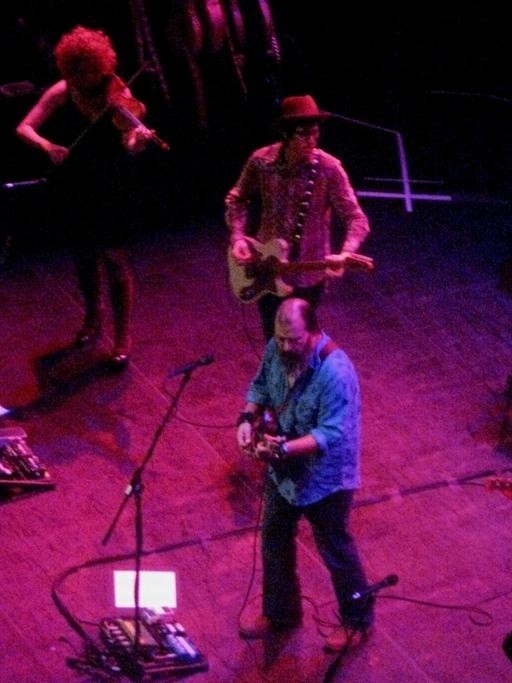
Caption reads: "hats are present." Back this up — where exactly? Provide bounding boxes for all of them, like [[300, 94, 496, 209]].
[[277, 93, 333, 120]]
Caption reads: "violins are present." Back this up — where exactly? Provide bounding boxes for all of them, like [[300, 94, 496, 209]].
[[68, 66, 172, 154]]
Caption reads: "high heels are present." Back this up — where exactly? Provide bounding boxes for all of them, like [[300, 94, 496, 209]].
[[76, 311, 108, 346], [108, 333, 132, 369]]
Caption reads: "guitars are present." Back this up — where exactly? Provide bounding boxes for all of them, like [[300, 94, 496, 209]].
[[228, 238, 373, 307], [239, 408, 286, 461]]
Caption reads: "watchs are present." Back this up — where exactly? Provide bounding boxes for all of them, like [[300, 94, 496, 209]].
[[238, 410, 253, 425]]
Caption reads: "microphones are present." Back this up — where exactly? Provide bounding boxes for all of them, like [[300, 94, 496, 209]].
[[167, 352, 216, 376], [351, 572, 400, 598]]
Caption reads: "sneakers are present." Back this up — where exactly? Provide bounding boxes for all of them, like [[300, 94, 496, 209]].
[[242, 613, 278, 638], [327, 621, 371, 649]]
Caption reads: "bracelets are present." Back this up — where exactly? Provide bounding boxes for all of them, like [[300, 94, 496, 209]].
[[274, 444, 288, 460], [238, 411, 252, 424]]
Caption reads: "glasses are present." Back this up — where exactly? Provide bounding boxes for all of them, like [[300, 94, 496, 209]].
[[292, 129, 322, 139]]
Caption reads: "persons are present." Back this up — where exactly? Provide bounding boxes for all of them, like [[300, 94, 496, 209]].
[[236, 297, 368, 654], [16, 25, 156, 363], [224, 95, 370, 342]]
